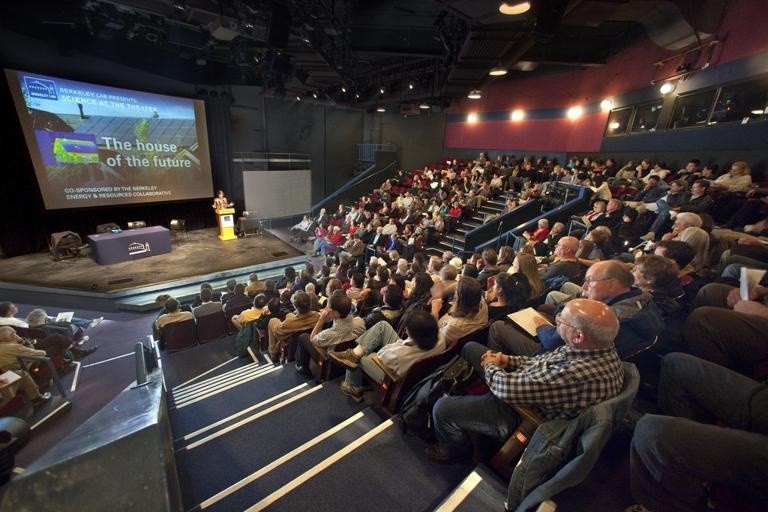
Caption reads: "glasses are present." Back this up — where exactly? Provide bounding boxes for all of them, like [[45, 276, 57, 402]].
[[555, 313, 575, 330], [584, 278, 613, 287]]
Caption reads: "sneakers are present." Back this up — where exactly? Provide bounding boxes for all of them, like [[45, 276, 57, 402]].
[[90, 316, 103, 328], [78, 335, 89, 346], [425, 448, 450, 463], [341, 381, 365, 403]]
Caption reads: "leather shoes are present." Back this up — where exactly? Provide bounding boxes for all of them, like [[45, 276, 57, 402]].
[[33, 392, 53, 409], [73, 346, 97, 360], [295, 364, 310, 378]]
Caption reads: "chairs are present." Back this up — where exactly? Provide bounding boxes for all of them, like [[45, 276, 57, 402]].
[[157, 319, 196, 350], [306, 338, 358, 380], [479, 365, 640, 470], [621, 335, 660, 360], [196, 312, 224, 345], [225, 303, 253, 335], [654, 290, 685, 308], [456, 319, 492, 345], [369, 343, 457, 409]]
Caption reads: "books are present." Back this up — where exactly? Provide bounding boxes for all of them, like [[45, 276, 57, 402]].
[[0, 369, 23, 390], [55, 311, 75, 324], [504, 304, 556, 344]]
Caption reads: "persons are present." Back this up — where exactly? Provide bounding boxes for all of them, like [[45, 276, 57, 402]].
[[476, 247, 501, 291], [638, 159, 672, 186], [719, 237, 768, 282], [537, 235, 580, 287], [512, 218, 550, 249], [486, 259, 664, 359], [697, 213, 719, 251], [595, 225, 611, 238], [191, 282, 222, 308], [152, 294, 195, 336], [221, 249, 443, 404], [508, 252, 545, 300], [654, 179, 688, 213], [661, 211, 703, 243], [639, 178, 714, 242], [543, 254, 679, 307], [0, 299, 29, 329], [720, 186, 768, 236], [674, 225, 710, 277], [211, 189, 234, 235], [0, 326, 101, 373], [288, 156, 394, 259], [615, 159, 636, 181], [461, 263, 480, 280], [421, 296, 626, 465], [589, 174, 612, 211], [576, 229, 613, 268], [587, 197, 624, 227], [438, 264, 458, 288], [442, 250, 454, 266], [621, 350, 768, 512], [496, 245, 516, 273], [519, 244, 535, 256], [563, 200, 606, 239], [590, 158, 606, 173], [465, 252, 482, 267], [0, 368, 52, 413], [487, 271, 533, 321], [632, 175, 668, 204], [601, 159, 618, 181], [527, 221, 566, 257], [574, 238, 595, 260], [672, 178, 713, 215], [486, 152, 589, 218], [686, 159, 720, 186], [634, 159, 651, 182], [449, 256, 463, 270], [193, 287, 223, 320], [612, 208, 638, 247], [688, 281, 768, 369], [25, 307, 105, 347], [671, 159, 702, 180], [653, 239, 698, 288], [712, 160, 753, 208], [442, 275, 489, 348], [393, 150, 486, 258]]
